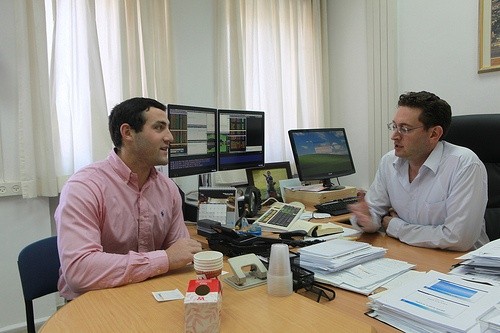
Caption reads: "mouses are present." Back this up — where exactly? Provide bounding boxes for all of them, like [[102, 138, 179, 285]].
[[289, 201, 306, 210]]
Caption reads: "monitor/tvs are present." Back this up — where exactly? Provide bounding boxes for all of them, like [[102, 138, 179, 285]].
[[288, 128, 356, 191], [165, 103, 265, 178]]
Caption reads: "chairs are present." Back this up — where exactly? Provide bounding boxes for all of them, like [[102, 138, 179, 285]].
[[18, 236, 60, 333], [444, 114, 500, 241]]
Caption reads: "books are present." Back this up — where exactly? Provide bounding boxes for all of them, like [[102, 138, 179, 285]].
[[287, 216, 343, 239]]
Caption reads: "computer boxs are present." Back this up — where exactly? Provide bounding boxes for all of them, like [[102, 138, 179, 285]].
[[284, 183, 358, 210]]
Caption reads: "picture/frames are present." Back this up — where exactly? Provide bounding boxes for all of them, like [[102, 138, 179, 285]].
[[478, 0, 500, 74], [246, 161, 293, 205]]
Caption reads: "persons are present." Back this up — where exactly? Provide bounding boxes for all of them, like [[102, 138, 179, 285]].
[[266, 170, 277, 191], [346, 90, 489, 252], [53, 97, 202, 305]]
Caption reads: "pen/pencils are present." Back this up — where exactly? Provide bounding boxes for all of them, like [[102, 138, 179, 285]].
[[449, 260, 466, 269]]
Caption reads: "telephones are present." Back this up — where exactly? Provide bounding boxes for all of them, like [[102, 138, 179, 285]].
[[198, 219, 282, 257]]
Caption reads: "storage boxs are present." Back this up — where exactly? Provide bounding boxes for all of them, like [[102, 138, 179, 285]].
[[183, 278, 222, 333]]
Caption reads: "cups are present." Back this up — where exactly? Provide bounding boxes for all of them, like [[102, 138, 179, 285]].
[[193, 250, 224, 281], [267, 243, 294, 297]]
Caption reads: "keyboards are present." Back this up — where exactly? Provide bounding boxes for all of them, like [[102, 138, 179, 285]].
[[252, 202, 303, 231], [313, 197, 358, 216]]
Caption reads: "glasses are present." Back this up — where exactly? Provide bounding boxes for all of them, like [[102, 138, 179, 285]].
[[387, 122, 424, 135]]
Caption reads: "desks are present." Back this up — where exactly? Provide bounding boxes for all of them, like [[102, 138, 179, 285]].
[[39, 206, 469, 333]]
